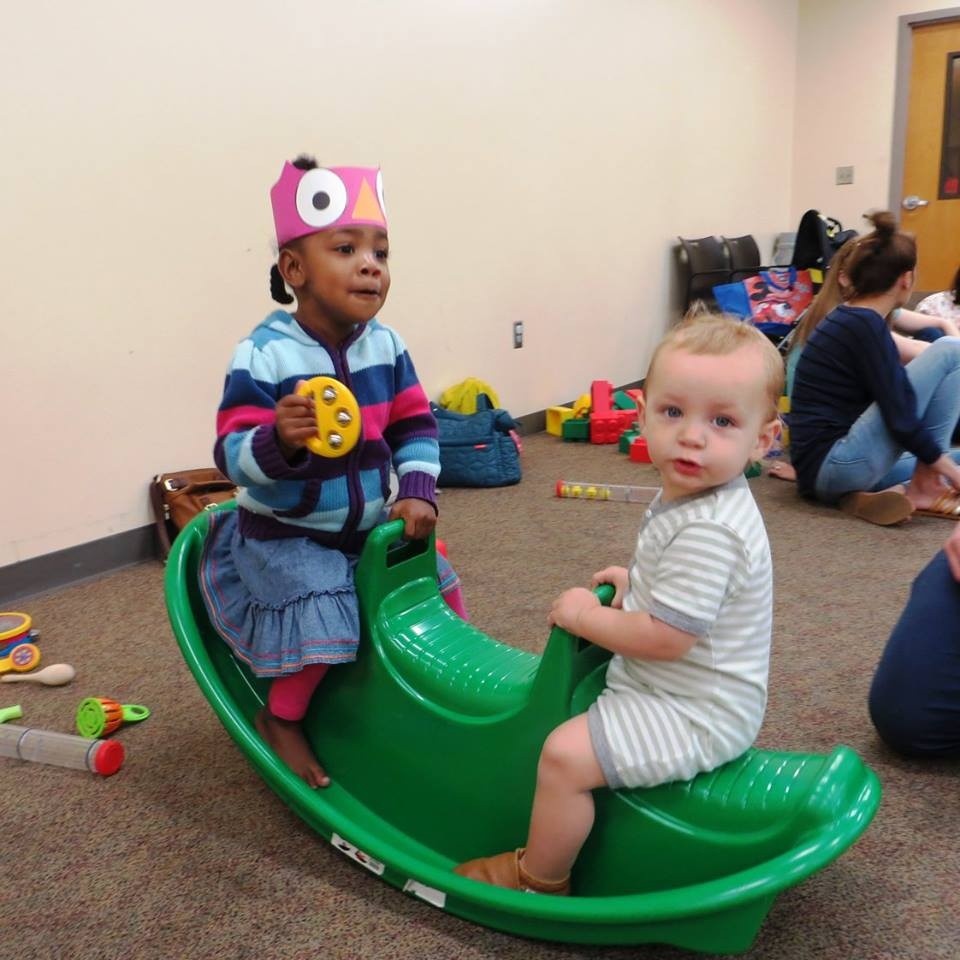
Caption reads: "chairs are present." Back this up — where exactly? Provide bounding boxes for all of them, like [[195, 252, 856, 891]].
[[679, 235, 791, 315]]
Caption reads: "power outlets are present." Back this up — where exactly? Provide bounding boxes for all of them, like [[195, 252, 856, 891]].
[[512, 321, 524, 349]]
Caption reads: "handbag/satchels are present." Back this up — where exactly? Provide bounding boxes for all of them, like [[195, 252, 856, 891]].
[[431, 393, 521, 487], [712, 265, 812, 335]]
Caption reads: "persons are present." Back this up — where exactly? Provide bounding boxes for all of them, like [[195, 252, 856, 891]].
[[451, 299, 784, 906], [868, 520, 960, 776], [788, 212, 960, 526], [198, 151, 464, 792]]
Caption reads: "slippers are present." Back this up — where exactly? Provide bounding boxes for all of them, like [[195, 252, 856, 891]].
[[908, 485, 960, 523], [844, 489, 913, 525]]
